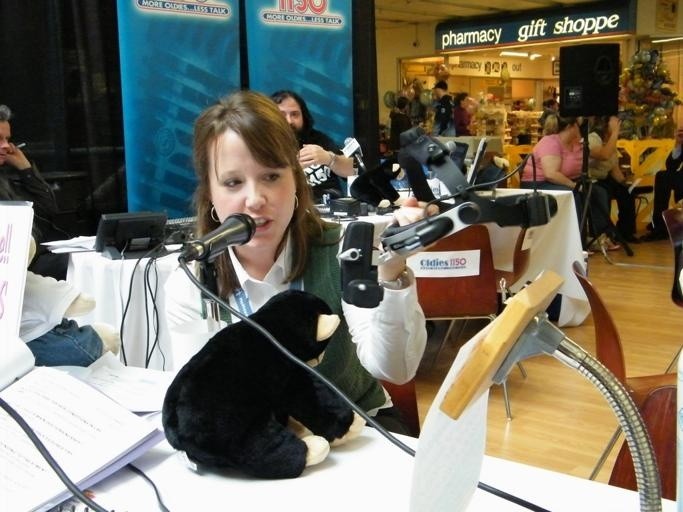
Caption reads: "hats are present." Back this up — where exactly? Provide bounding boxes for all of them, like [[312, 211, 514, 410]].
[[432, 80, 448, 90]]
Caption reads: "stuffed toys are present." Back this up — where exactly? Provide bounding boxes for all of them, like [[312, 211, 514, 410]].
[[350, 157, 405, 209], [158, 289, 364, 476]]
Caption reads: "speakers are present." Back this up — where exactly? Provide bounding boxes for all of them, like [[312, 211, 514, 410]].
[[558, 42, 621, 118]]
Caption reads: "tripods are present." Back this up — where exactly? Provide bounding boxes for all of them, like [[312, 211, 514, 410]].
[[571, 132, 634, 258]]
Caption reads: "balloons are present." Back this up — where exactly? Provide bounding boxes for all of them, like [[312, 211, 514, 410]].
[[383, 90, 396, 109], [434, 64, 450, 83], [616, 49, 678, 135]]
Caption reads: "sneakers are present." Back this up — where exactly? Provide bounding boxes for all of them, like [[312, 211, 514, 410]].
[[613, 234, 642, 244], [585, 234, 621, 256]]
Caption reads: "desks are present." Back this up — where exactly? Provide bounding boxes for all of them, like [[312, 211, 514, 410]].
[[0, 187, 677, 512], [433, 136, 504, 158]]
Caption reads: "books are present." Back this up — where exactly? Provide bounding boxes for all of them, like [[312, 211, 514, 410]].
[[0, 321, 164, 512]]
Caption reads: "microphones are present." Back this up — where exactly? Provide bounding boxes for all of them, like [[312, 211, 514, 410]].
[[176, 212, 257, 261], [340, 135, 369, 172], [445, 140, 458, 153]]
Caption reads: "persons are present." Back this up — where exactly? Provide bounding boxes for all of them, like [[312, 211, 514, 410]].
[[272, 92, 357, 201], [0, 104, 71, 282], [152, 90, 438, 436], [388, 79, 683, 256]]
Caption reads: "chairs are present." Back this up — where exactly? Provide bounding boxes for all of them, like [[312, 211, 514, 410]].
[[449, 136, 510, 190]]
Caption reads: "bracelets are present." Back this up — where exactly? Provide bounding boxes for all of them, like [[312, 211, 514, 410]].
[[327, 150, 336, 170]]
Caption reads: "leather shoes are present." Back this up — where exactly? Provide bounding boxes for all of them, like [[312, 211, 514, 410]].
[[640, 222, 670, 242]]
